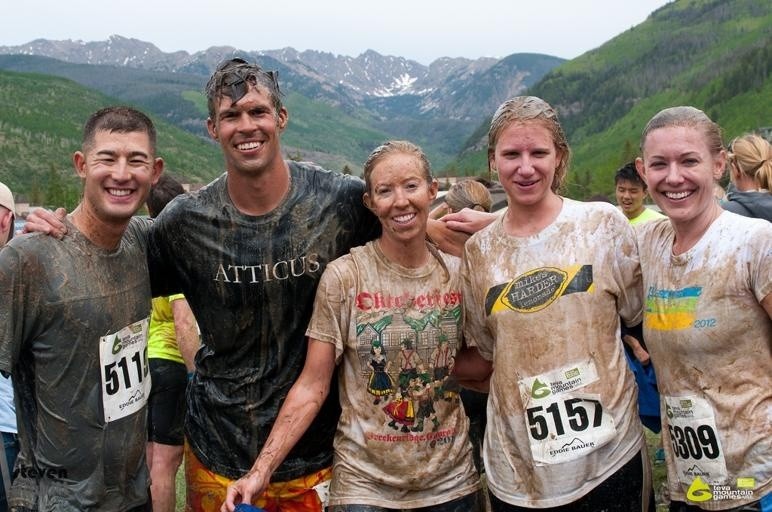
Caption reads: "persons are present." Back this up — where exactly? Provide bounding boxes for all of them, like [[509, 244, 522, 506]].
[[22, 53, 478, 512], [425, 177, 660, 433], [145, 167, 204, 510], [219, 135, 490, 511], [716, 132, 771, 221], [0, 104, 160, 512], [437, 98, 772, 509], [0, 183, 20, 512], [448, 92, 663, 511], [609, 160, 671, 229]]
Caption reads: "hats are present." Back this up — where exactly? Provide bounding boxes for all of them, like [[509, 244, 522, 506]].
[[0, 182, 17, 244]]
